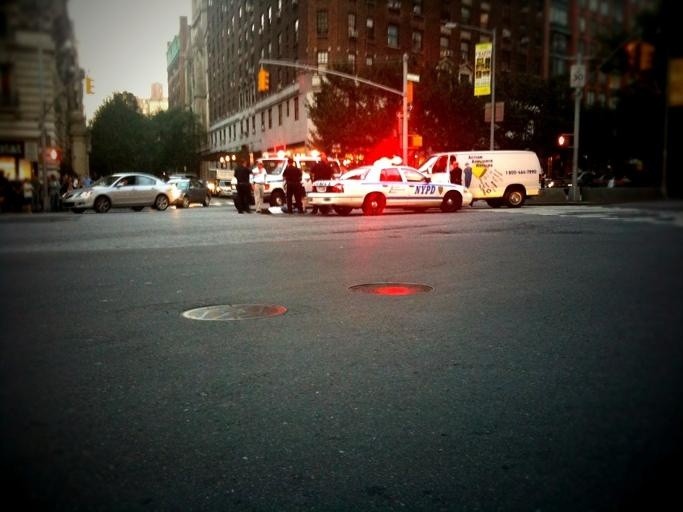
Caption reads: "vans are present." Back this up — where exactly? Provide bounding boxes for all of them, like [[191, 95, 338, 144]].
[[417, 149, 543, 208]]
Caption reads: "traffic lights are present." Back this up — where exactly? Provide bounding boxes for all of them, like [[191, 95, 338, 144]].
[[257, 70, 268, 93], [84, 76, 95, 95], [44, 147, 61, 161]]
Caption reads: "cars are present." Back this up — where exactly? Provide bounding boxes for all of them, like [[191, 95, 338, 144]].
[[60, 170, 174, 213], [167, 179, 210, 208], [306, 164, 474, 215], [214, 181, 231, 198], [230, 159, 309, 206]]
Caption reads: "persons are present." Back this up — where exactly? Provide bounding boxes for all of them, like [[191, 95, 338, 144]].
[[22, 174, 93, 215], [235, 152, 336, 214], [464, 163, 472, 188], [450, 161, 462, 185], [123, 179, 128, 184]]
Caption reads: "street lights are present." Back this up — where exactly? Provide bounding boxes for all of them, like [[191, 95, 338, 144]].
[[444, 20, 500, 151]]
